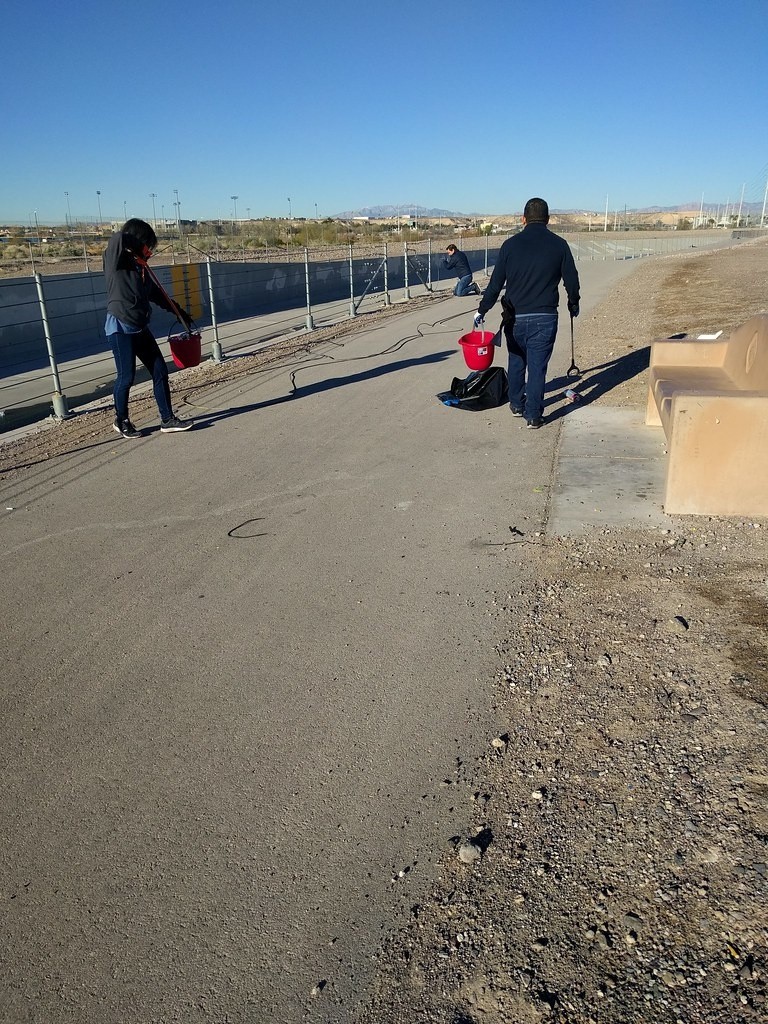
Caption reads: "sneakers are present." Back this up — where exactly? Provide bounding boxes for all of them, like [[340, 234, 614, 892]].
[[113, 416, 194, 439]]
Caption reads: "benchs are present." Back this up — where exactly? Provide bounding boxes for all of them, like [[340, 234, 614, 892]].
[[645, 313, 768, 518]]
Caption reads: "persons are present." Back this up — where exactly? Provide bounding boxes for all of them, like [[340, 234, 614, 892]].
[[442, 244, 481, 296], [104, 218, 194, 441], [472, 197, 582, 428]]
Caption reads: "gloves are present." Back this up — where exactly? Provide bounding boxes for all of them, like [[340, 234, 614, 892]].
[[473, 311, 485, 328], [567, 297, 581, 317]]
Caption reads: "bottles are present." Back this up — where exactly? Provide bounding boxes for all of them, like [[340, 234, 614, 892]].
[[564, 388, 581, 403]]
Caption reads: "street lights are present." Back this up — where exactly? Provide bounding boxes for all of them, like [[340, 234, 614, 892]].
[[28, 187, 324, 241]]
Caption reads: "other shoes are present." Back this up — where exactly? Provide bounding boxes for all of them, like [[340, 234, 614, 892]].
[[472, 282, 481, 295], [509, 401, 546, 429]]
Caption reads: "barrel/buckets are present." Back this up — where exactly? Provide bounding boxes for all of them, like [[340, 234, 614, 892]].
[[457, 316, 496, 371], [168, 318, 201, 370]]
